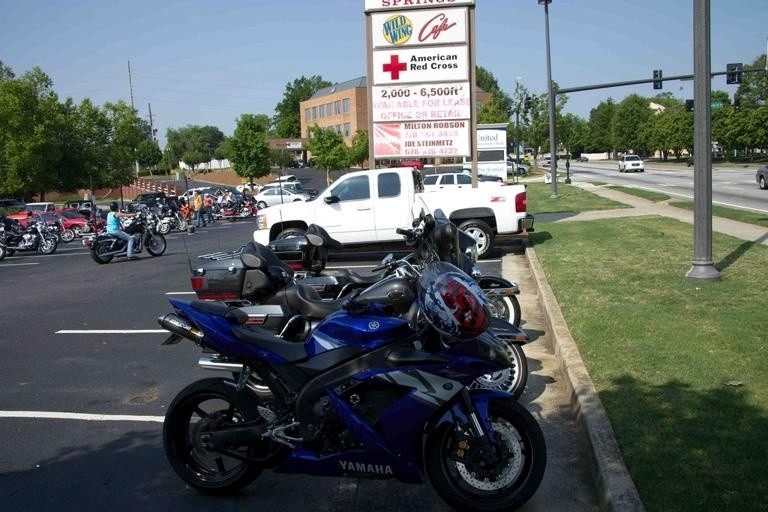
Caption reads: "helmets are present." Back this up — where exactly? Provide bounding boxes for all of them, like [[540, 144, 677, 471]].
[[110, 202, 118, 211], [417, 262, 491, 340]]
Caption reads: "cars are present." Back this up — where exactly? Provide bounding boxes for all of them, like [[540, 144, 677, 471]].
[[542, 156, 551, 167], [687, 154, 694, 167], [506, 160, 530, 177], [617, 154, 644, 173], [576, 157, 587, 162], [755, 163, 768, 189], [128, 175, 315, 223], [6, 200, 107, 239]]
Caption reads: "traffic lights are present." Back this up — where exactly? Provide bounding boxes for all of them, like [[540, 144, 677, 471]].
[[526, 96, 532, 109], [686, 99, 695, 112]]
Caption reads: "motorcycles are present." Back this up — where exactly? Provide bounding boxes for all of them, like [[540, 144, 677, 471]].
[[0, 211, 75, 262], [79, 197, 188, 249], [187, 194, 531, 400], [160, 295, 548, 510], [84, 212, 167, 264], [262, 207, 522, 331]]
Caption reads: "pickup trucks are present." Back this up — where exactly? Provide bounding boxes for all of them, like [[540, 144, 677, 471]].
[[252, 158, 535, 262]]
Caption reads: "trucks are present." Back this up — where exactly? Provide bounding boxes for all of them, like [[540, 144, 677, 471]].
[[711, 141, 723, 158]]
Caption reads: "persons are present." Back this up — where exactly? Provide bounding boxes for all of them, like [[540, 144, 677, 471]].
[[106, 201, 140, 261], [177, 188, 257, 227]]
